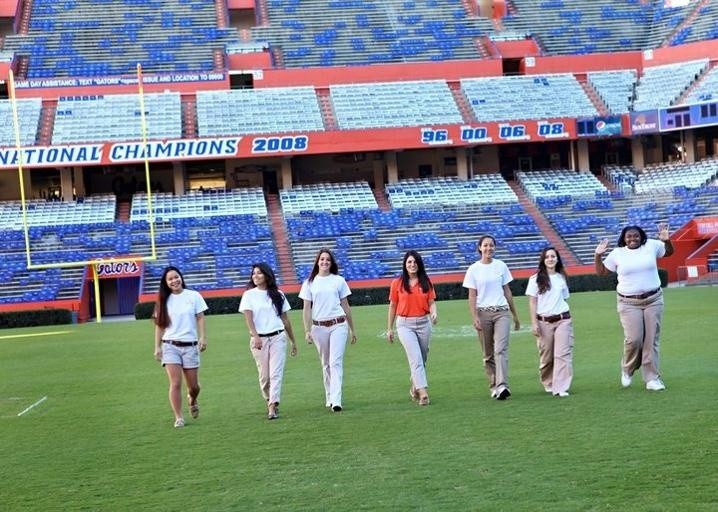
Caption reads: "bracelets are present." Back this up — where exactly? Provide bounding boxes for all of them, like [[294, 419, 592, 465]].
[[386, 328, 394, 335], [303, 328, 310, 334]]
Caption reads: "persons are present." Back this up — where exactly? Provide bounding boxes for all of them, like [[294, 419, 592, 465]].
[[151, 265, 210, 428], [461, 235, 521, 401], [297, 246, 358, 412], [386, 248, 439, 406], [524, 246, 575, 398], [594, 221, 676, 393], [238, 261, 297, 420]]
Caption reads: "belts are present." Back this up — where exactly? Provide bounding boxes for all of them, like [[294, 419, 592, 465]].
[[161, 341, 197, 346], [536, 311, 571, 324], [476, 305, 508, 313], [258, 330, 283, 337], [313, 318, 344, 327], [617, 288, 659, 299]]
